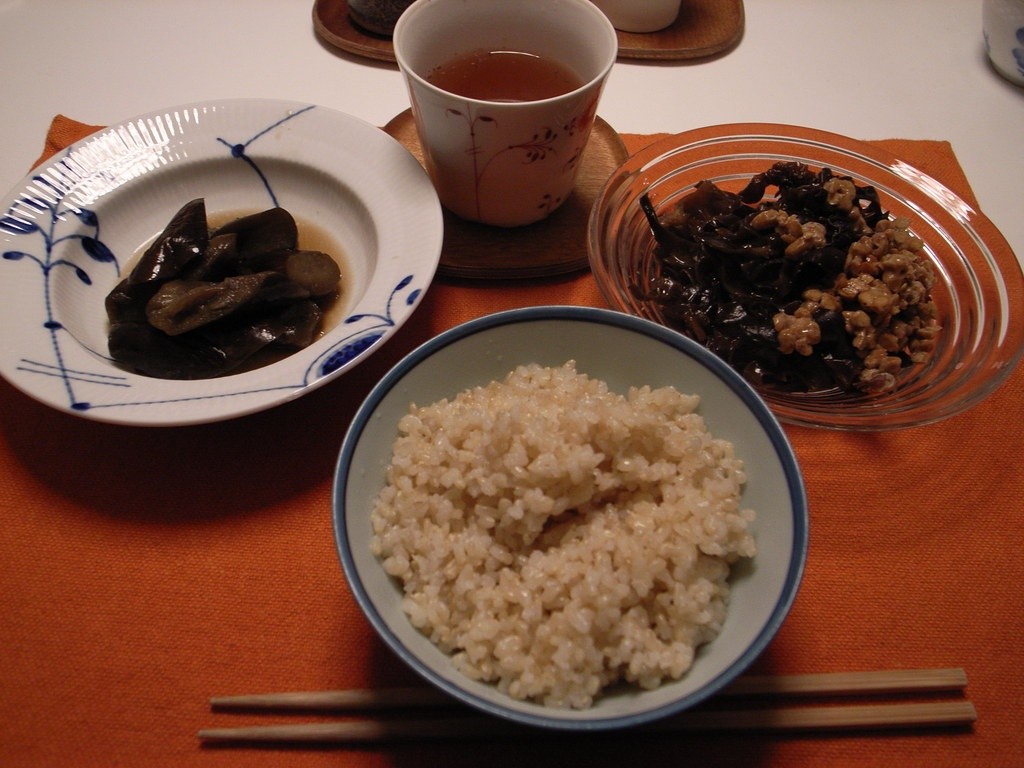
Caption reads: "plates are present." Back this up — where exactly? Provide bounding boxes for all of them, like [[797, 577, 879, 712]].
[[0, 95, 443, 427]]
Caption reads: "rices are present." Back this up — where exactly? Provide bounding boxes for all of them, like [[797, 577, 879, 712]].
[[370, 360, 756, 709]]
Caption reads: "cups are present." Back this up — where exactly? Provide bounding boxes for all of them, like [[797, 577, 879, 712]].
[[981, 0, 1024, 89], [589, 0, 682, 34], [347, 0, 416, 40], [393, 0, 618, 229]]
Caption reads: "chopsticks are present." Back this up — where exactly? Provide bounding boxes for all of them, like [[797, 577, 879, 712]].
[[197, 668, 979, 741]]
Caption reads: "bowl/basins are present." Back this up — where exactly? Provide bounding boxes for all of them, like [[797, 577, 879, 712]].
[[331, 303, 808, 733], [586, 122, 1024, 432]]
